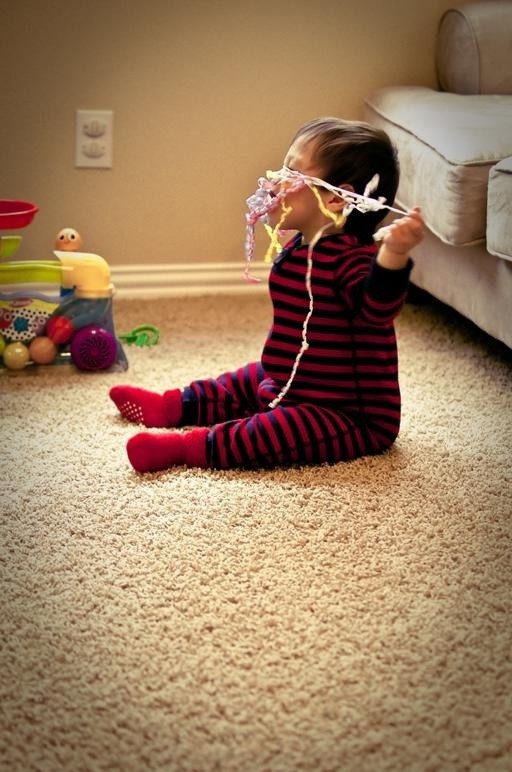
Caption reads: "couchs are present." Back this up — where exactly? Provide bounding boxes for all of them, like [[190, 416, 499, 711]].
[[364, 6, 512, 362]]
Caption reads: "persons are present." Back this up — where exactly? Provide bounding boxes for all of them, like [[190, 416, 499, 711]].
[[109, 115, 426, 473]]
